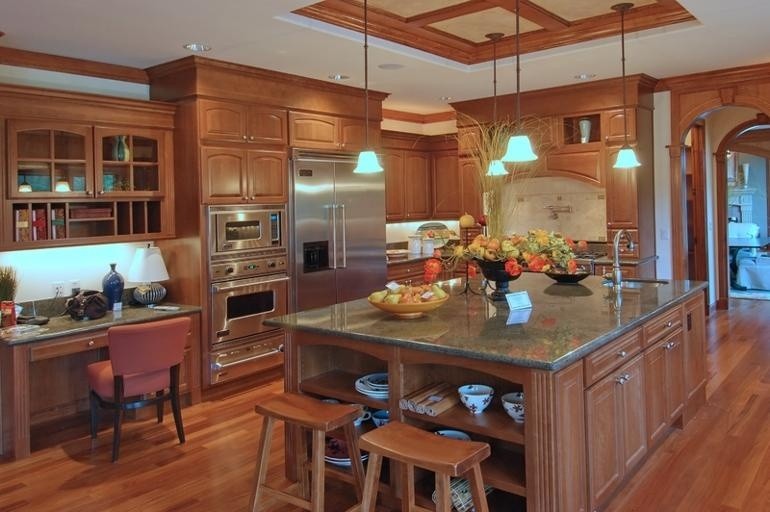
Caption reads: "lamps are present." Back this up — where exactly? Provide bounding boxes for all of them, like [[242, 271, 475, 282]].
[[126, 244, 170, 308], [501, 0, 538, 163], [485, 32, 509, 176], [610, 3, 642, 168], [352, 0, 384, 174]]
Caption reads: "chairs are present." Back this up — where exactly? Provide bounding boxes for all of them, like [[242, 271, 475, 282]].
[[87, 316, 191, 464]]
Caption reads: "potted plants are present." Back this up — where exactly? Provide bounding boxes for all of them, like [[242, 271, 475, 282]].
[[1, 266, 17, 327]]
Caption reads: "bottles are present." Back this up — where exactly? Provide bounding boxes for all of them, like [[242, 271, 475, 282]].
[[408, 235, 423, 255], [422, 239, 435, 255]]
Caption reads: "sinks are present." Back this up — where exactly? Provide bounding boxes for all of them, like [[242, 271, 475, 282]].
[[602, 280, 668, 289]]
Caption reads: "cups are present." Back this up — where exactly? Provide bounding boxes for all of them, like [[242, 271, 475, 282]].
[[104, 174, 115, 191], [350, 404, 371, 427], [72, 175, 86, 192]]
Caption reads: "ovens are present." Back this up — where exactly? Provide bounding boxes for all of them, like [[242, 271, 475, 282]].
[[206, 204, 290, 387]]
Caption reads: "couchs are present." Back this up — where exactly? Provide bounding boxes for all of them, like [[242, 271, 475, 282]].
[[728, 222, 760, 264]]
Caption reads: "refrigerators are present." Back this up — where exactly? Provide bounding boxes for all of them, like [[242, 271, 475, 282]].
[[288, 149, 388, 380]]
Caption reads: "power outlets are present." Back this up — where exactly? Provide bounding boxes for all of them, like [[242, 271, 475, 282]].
[[52, 282, 65, 295], [71, 279, 82, 294]]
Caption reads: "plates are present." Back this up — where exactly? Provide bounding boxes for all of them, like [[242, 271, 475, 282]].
[[354, 373, 389, 399], [435, 430, 471, 440], [324, 454, 368, 466], [386, 249, 411, 258]]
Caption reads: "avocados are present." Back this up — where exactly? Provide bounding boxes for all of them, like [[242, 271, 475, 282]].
[[369, 290, 388, 303], [431, 285, 446, 299], [383, 294, 402, 304]]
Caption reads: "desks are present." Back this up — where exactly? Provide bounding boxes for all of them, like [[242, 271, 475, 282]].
[[728, 237, 770, 291], [0, 302, 202, 461]]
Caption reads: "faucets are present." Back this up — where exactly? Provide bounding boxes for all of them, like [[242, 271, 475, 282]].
[[613, 229, 634, 287], [611, 287, 623, 328]]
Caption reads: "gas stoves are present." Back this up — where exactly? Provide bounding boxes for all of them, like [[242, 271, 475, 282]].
[[574, 250, 608, 260]]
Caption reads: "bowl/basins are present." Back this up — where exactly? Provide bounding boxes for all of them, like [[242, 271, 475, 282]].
[[500, 391, 527, 423], [544, 271, 591, 283], [457, 383, 495, 414], [367, 314, 450, 339], [24, 175, 62, 192], [371, 410, 391, 427], [368, 293, 449, 319], [544, 283, 593, 297]]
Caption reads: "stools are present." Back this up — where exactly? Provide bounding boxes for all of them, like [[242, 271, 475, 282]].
[[247, 392, 366, 512]]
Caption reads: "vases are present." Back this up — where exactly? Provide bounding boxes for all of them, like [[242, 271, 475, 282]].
[[102, 263, 124, 311]]
